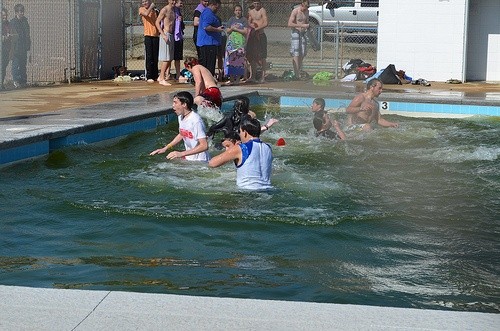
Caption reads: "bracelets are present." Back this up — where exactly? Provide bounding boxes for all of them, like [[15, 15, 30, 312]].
[[168, 144, 172, 149], [264, 125, 268, 130]]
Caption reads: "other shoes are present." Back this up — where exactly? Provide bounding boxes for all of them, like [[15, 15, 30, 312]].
[[411, 78, 431, 87]]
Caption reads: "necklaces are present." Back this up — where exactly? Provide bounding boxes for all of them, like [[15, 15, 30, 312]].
[[181, 110, 192, 120]]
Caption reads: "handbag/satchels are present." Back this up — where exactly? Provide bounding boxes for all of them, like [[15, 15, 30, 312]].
[[312, 71, 334, 81], [342, 57, 376, 80]]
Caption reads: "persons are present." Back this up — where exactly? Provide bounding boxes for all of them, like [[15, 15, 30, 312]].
[[156, 0, 185, 86], [221, 132, 241, 151], [150, 91, 208, 161], [139, 0, 160, 83], [346, 78, 399, 132], [224, 0, 268, 85], [0, 4, 31, 90], [184, 56, 222, 109], [207, 96, 278, 142], [288, 0, 310, 80], [311, 97, 345, 140], [209, 117, 273, 189], [193, 0, 224, 78]]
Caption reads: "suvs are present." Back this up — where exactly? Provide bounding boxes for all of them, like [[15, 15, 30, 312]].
[[306, 0, 379, 42]]
